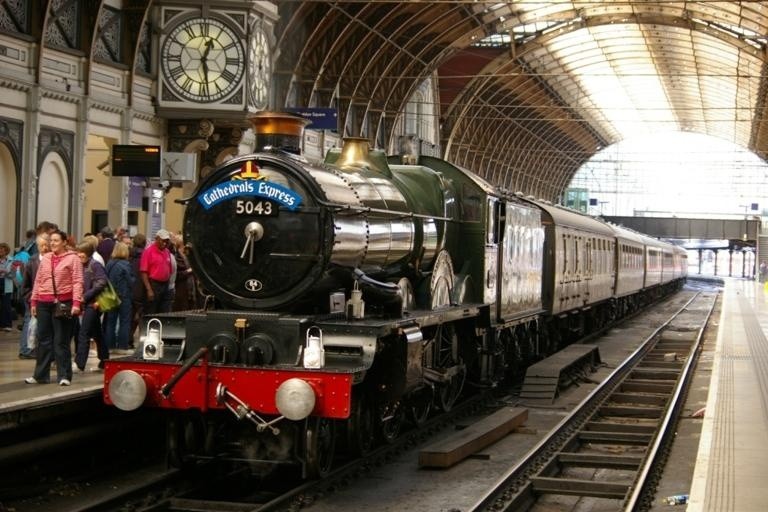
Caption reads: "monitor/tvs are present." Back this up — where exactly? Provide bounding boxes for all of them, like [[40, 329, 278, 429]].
[[150, 151, 197, 182], [111, 144, 162, 178]]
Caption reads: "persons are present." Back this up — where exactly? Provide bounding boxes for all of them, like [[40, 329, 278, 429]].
[[2, 220, 212, 371], [23, 229, 86, 388]]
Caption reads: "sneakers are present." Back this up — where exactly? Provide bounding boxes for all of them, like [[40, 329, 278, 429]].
[[25, 377, 37, 384], [72, 362, 104, 372], [60, 379, 70, 386]]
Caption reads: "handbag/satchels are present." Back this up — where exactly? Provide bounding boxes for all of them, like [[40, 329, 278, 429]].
[[26, 316, 38, 349], [97, 280, 122, 313], [55, 303, 73, 322]]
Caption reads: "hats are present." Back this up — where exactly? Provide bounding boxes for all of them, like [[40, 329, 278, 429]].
[[156, 229, 170, 240]]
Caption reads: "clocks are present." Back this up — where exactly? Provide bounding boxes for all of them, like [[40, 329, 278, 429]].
[[151, 0, 281, 122]]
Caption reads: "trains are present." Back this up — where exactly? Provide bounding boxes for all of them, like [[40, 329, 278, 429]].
[[100, 108, 689, 483]]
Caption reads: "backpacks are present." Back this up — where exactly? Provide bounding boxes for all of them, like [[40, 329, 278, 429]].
[[8, 242, 34, 287]]
